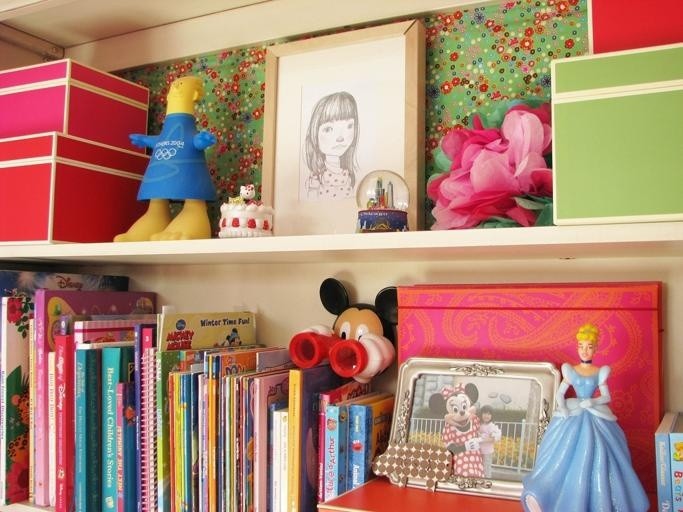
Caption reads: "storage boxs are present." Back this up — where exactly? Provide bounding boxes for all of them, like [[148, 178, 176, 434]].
[[586, 1, 682, 56], [0, 59, 151, 155], [0, 132, 152, 242], [550, 42, 682, 227]]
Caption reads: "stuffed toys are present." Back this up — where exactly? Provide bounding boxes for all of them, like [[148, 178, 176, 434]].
[[428, 383, 492, 487]]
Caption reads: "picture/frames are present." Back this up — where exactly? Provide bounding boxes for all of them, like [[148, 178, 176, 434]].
[[260, 18, 425, 237], [373, 356, 561, 501]]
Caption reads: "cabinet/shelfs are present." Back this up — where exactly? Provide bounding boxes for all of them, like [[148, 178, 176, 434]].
[[0, 0, 682, 512]]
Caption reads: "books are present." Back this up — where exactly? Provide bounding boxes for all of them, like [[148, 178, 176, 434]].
[[670, 411, 683, 511], [656, 410, 678, 511], [0, 270, 396, 511]]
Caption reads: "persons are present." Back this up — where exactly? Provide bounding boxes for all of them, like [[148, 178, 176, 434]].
[[115, 74, 219, 241], [517, 322, 653, 511], [477, 403, 502, 479], [300, 90, 359, 200]]
